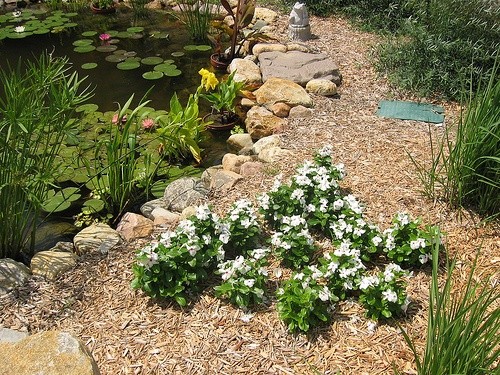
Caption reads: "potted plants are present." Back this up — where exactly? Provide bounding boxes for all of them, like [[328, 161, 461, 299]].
[[209, 1, 257, 72], [90, 0, 117, 15], [201, 69, 249, 133]]
